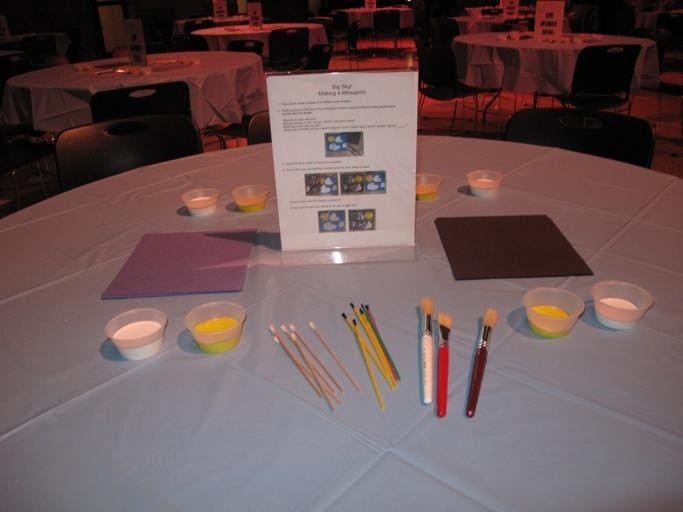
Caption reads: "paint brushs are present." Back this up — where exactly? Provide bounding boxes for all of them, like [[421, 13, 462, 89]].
[[419, 298, 452, 419], [269, 321, 360, 412], [466, 307, 498, 417], [341, 302, 402, 410]]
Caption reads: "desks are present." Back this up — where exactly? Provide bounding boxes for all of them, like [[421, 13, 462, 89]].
[[191, 23, 328, 57], [5, 51, 269, 129], [0, 134, 682, 512], [449, 5, 537, 31], [451, 32, 657, 122]]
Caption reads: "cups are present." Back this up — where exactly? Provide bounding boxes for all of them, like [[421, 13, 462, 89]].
[[185, 302, 247, 352], [230, 184, 270, 213], [589, 279, 654, 330], [519, 287, 584, 336], [510, 31, 519, 41], [466, 169, 503, 198], [104, 307, 166, 361], [181, 188, 220, 217], [414, 174, 442, 201]]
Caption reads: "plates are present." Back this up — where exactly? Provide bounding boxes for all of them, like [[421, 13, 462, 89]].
[[151, 59, 193, 70], [95, 58, 129, 67]]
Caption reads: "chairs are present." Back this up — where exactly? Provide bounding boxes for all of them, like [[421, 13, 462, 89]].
[[329, 10, 414, 56], [2, 28, 105, 60], [503, 108, 655, 169], [248, 111, 271, 144], [167, 21, 217, 51], [415, 4, 484, 132], [2, 125, 55, 210], [54, 81, 203, 192], [227, 29, 334, 72], [554, 4, 680, 125]]
